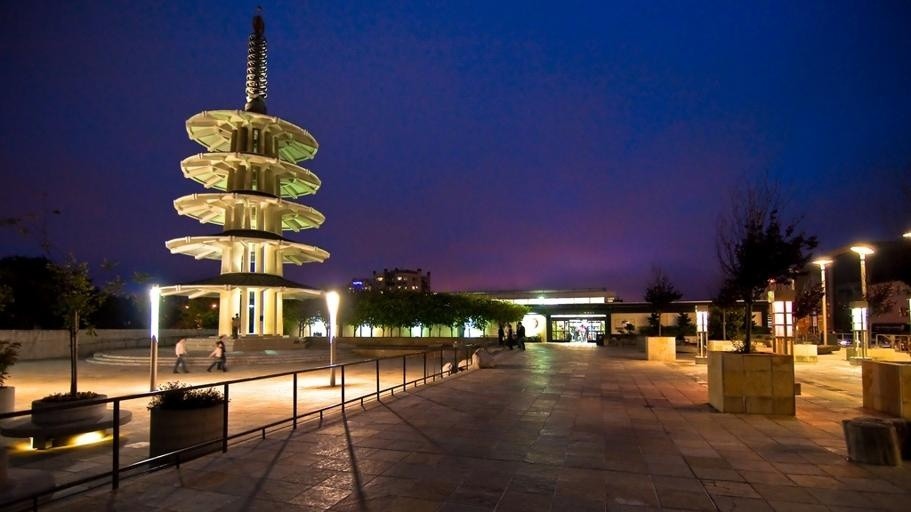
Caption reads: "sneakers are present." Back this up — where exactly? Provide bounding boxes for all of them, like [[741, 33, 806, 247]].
[[173, 369, 191, 373], [231, 334, 238, 340], [208, 367, 229, 373]]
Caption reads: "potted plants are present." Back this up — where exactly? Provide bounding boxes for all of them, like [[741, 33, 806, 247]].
[[1, 337, 226, 456]]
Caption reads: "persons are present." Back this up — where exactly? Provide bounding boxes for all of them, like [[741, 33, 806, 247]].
[[234, 313, 240, 338], [217, 336, 228, 370], [569, 324, 588, 342], [231, 317, 237, 339], [518, 322, 526, 350], [498, 325, 505, 346], [507, 324, 514, 349], [207, 341, 228, 372], [173, 336, 190, 373]]
